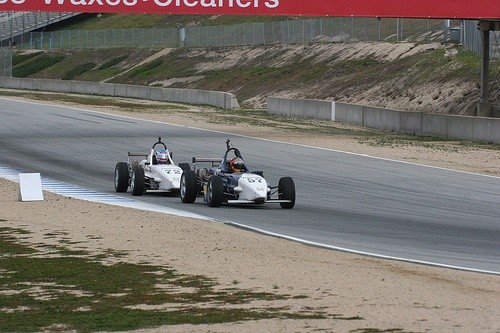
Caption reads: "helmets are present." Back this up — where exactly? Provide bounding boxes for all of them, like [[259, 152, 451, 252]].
[[230, 157, 246, 172], [156, 149, 168, 162]]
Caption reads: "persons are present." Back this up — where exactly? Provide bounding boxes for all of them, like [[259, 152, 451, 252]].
[[153, 149, 169, 165], [228, 156, 245, 175]]
